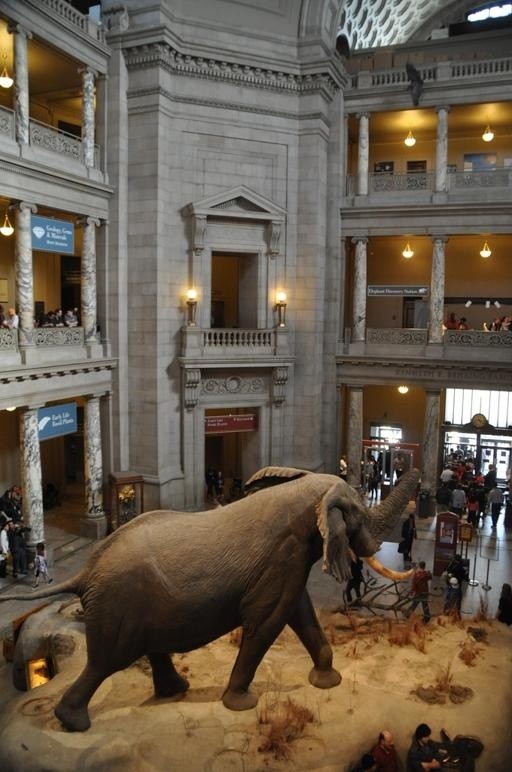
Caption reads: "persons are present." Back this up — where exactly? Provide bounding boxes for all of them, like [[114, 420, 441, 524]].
[[0, 486, 56, 590], [1, 303, 78, 331], [206, 465, 225, 501], [352, 750, 377, 771], [370, 730, 400, 771], [406, 723, 444, 772], [415, 300, 431, 329], [338, 445, 511, 622], [445, 312, 512, 331]]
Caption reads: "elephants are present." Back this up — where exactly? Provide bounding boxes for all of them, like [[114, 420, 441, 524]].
[[0, 465, 421, 733]]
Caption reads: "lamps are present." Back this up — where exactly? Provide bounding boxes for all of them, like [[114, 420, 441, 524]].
[[0, 54, 14, 88], [276, 292, 288, 326], [479, 241, 491, 257], [403, 125, 416, 146], [401, 241, 412, 257], [0, 213, 15, 235], [186, 288, 199, 326]]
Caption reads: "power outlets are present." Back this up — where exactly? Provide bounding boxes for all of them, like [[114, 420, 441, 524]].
[[481, 124, 494, 143]]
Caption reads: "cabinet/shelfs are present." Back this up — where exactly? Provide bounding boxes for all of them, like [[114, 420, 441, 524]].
[[107, 470, 143, 536]]
